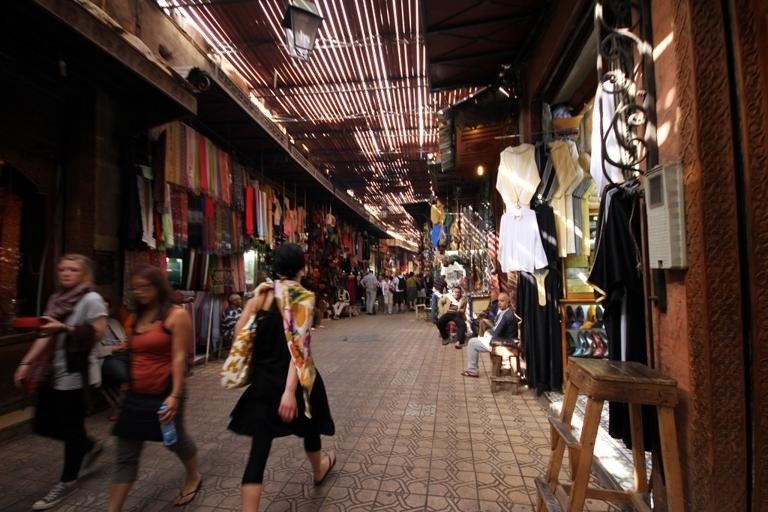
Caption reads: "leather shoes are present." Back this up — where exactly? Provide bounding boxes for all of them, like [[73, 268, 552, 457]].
[[565, 305, 609, 358]]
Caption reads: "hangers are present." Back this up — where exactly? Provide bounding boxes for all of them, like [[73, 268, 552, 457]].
[[505, 129, 568, 148]]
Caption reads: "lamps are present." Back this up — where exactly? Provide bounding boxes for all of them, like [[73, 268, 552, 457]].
[[281, 0, 324, 61]]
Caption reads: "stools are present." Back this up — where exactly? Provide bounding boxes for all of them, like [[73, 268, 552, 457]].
[[534, 357, 685, 512], [340, 305, 351, 319], [415, 304, 432, 320], [490, 338, 519, 395], [446, 321, 458, 341]]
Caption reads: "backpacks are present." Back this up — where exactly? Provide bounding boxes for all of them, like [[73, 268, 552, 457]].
[[397, 276, 407, 290]]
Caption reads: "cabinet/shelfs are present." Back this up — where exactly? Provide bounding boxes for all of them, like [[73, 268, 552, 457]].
[[560, 299, 609, 393]]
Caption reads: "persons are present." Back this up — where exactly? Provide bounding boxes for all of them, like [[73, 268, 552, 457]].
[[461, 292, 516, 377], [220, 294, 244, 340], [106, 264, 202, 511], [436, 286, 467, 348], [361, 268, 434, 315], [226, 242, 336, 512], [86, 298, 130, 386], [13, 255, 109, 512], [332, 286, 351, 320], [430, 275, 446, 322]]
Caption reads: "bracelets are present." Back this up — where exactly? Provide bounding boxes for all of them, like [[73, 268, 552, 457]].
[[171, 391, 183, 400], [18, 360, 32, 366]]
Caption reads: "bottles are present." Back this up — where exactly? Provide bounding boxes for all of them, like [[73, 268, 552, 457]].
[[159, 405, 178, 446]]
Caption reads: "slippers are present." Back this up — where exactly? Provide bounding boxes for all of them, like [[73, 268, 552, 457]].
[[460, 370, 479, 377], [174, 478, 202, 507], [314, 452, 336, 486]]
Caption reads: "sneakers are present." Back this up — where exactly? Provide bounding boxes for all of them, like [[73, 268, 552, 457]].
[[443, 338, 449, 345], [455, 340, 463, 349], [31, 480, 80, 510], [78, 443, 105, 478]]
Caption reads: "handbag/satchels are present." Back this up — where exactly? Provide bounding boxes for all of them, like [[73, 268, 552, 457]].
[[219, 296, 260, 390]]
